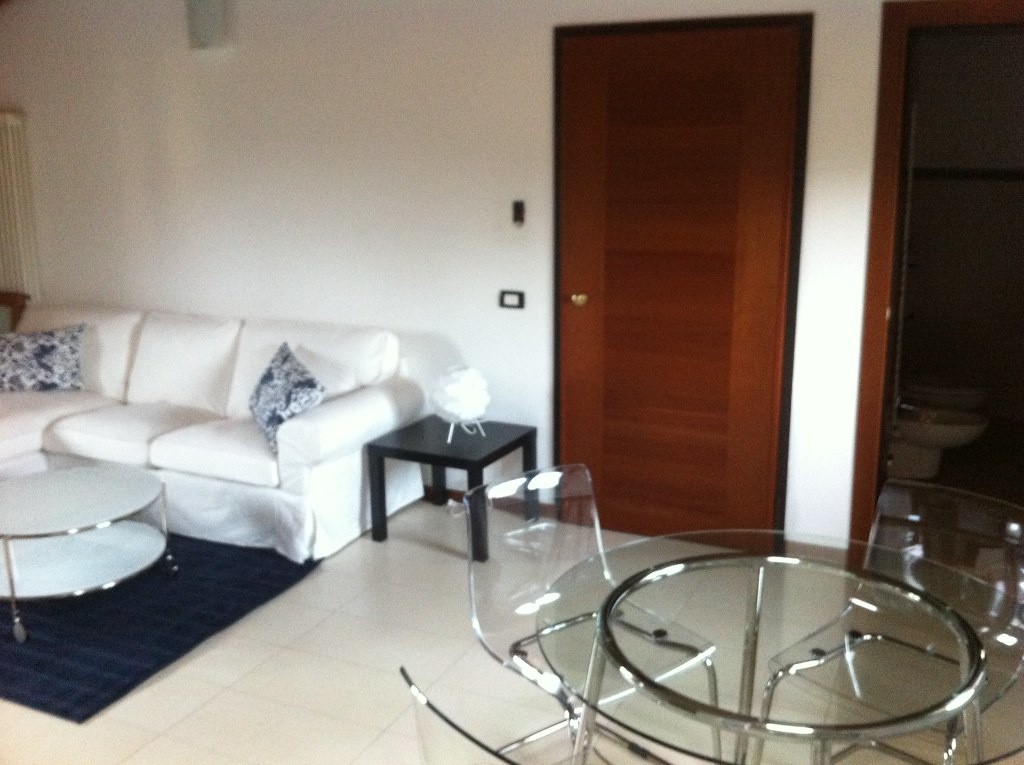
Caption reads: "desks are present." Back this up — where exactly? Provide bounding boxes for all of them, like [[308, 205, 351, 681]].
[[536, 530, 1023, 765]]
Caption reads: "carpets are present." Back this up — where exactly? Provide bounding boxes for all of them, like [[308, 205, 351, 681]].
[[1, 531, 322, 724]]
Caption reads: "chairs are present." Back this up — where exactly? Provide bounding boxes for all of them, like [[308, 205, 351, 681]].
[[400, 462, 1023, 765]]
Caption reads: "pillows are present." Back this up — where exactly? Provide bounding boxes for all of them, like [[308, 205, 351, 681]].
[[250, 342, 325, 458], [1, 322, 88, 394]]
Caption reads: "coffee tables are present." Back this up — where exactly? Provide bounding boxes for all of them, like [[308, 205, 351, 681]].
[[1, 463, 178, 645]]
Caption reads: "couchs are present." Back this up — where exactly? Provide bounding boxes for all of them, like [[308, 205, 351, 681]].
[[0, 306, 425, 563]]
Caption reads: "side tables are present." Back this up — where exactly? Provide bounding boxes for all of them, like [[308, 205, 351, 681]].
[[365, 414, 539, 562]]
